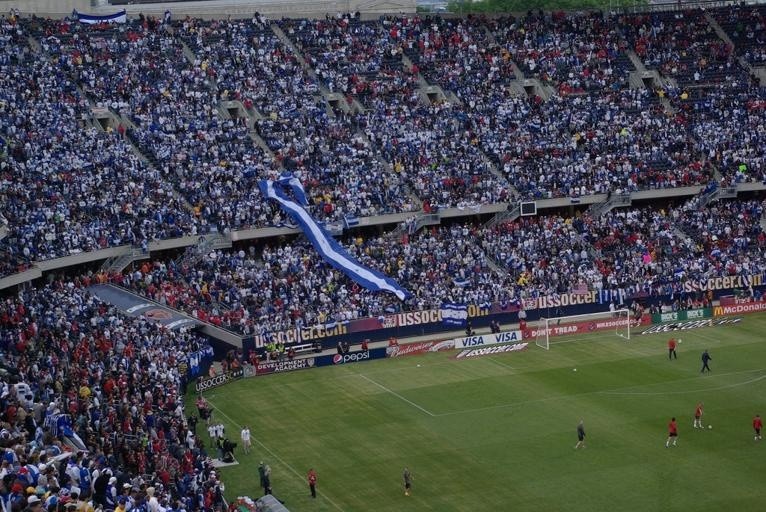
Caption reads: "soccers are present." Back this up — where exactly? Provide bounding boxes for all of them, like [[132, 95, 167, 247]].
[[678, 339, 682, 344], [574, 368, 576, 371], [709, 425, 712, 429]]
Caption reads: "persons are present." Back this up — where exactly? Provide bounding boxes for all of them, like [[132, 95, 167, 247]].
[[241, 425, 251, 455], [221, 10, 371, 371], [258, 460, 264, 487], [753, 413, 763, 440], [264, 468, 272, 495], [668, 336, 677, 359], [693, 402, 705, 429], [701, 350, 712, 372], [1, 8, 238, 512], [402, 466, 412, 495], [308, 467, 317, 498], [371, 0, 765, 346], [666, 416, 679, 448], [576, 417, 585, 448]]
